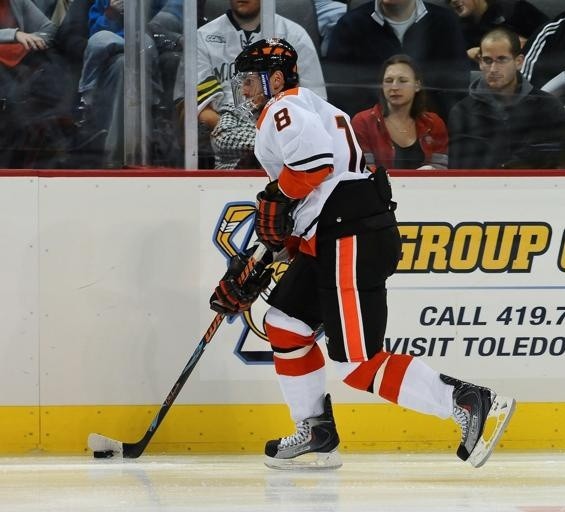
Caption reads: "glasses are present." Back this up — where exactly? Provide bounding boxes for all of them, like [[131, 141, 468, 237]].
[[478, 54, 513, 65]]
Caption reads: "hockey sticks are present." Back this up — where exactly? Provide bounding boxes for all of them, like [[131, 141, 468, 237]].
[[88, 243, 276, 459]]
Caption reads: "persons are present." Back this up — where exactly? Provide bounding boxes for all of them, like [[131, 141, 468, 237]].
[[209, 35, 497, 462], [0, 0, 565, 173]]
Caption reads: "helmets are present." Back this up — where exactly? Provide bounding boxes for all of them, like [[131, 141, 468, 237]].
[[231, 37, 299, 108]]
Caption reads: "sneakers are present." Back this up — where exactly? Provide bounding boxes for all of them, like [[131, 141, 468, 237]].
[[265, 393, 340, 459], [440, 373, 497, 461], [64, 125, 107, 154]]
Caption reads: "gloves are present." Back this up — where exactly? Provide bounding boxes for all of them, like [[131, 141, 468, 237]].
[[254, 178, 294, 253], [209, 259, 275, 316]]
